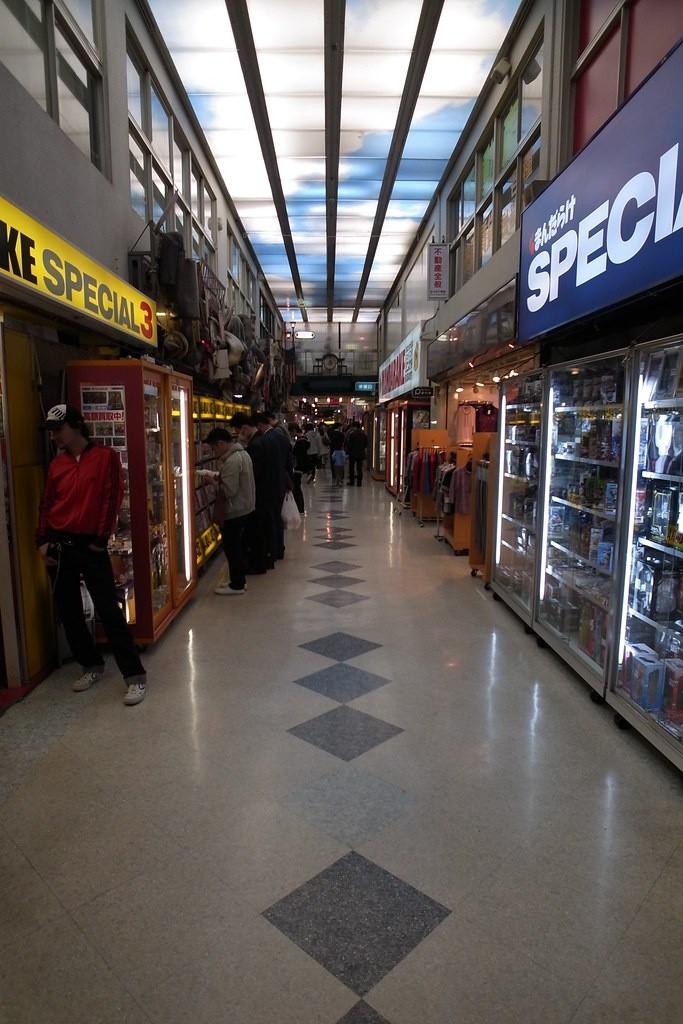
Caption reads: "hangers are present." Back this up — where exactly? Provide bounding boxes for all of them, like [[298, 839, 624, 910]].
[[462, 400, 494, 409], [410, 444, 489, 475]]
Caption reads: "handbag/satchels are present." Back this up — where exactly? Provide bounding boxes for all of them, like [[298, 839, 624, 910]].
[[280, 491, 302, 530], [212, 485, 226, 526]]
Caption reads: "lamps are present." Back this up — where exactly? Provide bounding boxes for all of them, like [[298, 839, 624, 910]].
[[282, 332, 291, 339]]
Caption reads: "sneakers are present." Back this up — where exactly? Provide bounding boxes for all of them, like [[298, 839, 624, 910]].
[[72, 671, 105, 691], [123, 683, 146, 705]]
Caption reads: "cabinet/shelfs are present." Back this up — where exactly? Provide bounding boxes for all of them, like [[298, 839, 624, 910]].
[[487, 333, 683, 775], [65, 362, 254, 647]]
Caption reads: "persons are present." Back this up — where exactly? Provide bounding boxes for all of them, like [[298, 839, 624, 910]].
[[202, 427, 256, 595], [249, 413, 293, 559], [286, 422, 310, 516], [233, 416, 275, 575], [230, 412, 246, 427], [300, 421, 369, 488], [35, 403, 149, 708], [262, 410, 296, 550]]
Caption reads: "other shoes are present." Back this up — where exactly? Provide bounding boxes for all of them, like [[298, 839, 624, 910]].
[[347, 482, 354, 486], [214, 581, 248, 594], [307, 474, 313, 484]]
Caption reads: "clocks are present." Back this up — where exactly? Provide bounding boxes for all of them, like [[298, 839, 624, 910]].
[[323, 355, 337, 370]]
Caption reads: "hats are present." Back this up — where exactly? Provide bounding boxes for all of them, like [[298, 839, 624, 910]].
[[44, 403, 78, 430]]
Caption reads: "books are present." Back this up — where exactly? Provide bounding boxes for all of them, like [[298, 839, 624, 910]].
[[80, 385, 240, 624]]
